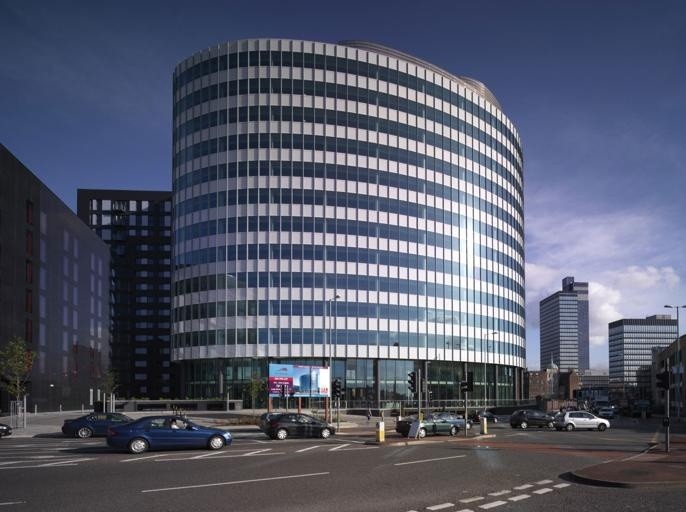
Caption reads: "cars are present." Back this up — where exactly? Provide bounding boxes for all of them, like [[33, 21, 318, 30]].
[[426, 411, 473, 432], [509, 405, 617, 433], [105, 416, 232, 455], [0, 423, 13, 438], [463, 409, 498, 424], [395, 414, 459, 439], [259, 411, 279, 432], [60, 413, 136, 440], [266, 413, 335, 440]]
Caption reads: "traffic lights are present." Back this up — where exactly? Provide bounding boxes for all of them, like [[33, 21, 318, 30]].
[[279, 384, 285, 400], [460, 380, 467, 393], [407, 372, 417, 393], [662, 372, 670, 391], [334, 380, 340, 397]]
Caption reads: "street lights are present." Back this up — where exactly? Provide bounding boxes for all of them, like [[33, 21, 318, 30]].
[[663, 305, 686, 420], [482, 331, 500, 410], [328, 295, 341, 368]]
